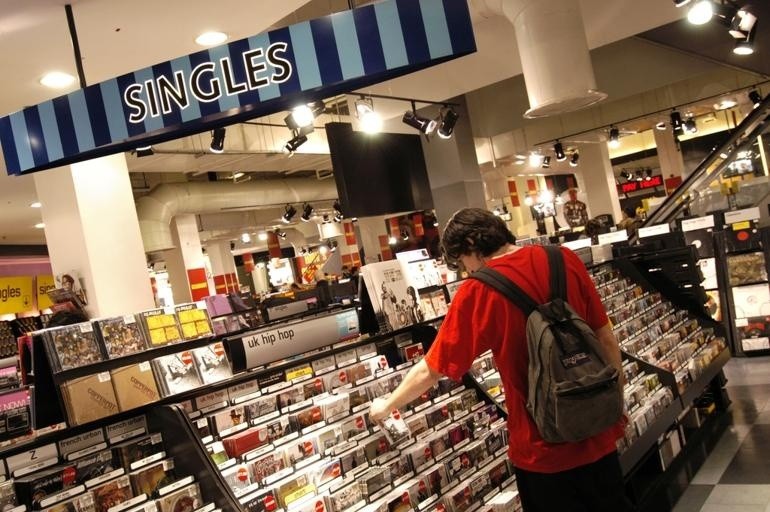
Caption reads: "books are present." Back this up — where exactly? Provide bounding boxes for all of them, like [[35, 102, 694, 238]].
[[0, 242, 731, 511]]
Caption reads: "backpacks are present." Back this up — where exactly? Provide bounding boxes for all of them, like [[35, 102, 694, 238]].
[[467, 245, 623, 445]]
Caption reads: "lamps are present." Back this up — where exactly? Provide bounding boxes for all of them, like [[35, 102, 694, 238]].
[[747, 88, 762, 108], [672, 0, 761, 55], [620, 166, 652, 182], [669, 108, 697, 137], [281, 91, 460, 157], [130, 141, 158, 160], [608, 124, 621, 148], [208, 125, 231, 155], [541, 139, 579, 169]]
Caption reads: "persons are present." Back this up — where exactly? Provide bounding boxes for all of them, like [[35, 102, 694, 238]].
[[367, 207, 634, 512]]
